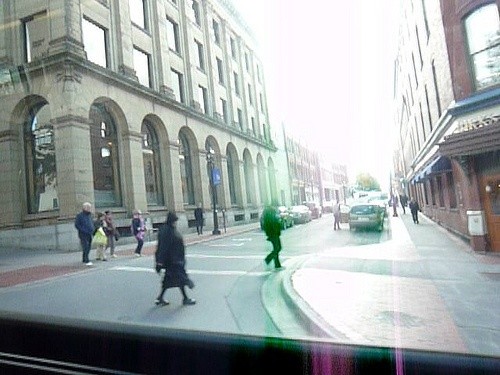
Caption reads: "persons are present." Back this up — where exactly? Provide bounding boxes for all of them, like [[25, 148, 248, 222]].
[[194, 203, 203, 235], [75, 202, 94, 265], [409, 196, 419, 224], [93, 213, 110, 261], [132, 209, 148, 256], [104, 211, 117, 258], [333, 201, 340, 230], [400, 195, 408, 214], [45, 170, 58, 199], [155, 211, 196, 306], [263, 199, 285, 270]]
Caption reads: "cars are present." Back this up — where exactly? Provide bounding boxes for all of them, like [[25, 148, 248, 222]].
[[260, 207, 295, 229], [321, 190, 388, 232], [302, 202, 323, 220], [286, 205, 312, 224]]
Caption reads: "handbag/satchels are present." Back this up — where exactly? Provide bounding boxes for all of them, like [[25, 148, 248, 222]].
[[92, 227, 107, 245]]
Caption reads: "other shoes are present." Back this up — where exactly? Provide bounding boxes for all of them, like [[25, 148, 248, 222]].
[[85, 262, 93, 266], [134, 253, 145, 257], [96, 258, 101, 260], [265, 257, 271, 264], [103, 259, 108, 261], [275, 262, 281, 267], [184, 297, 195, 304], [111, 254, 117, 257], [155, 299, 169, 306]]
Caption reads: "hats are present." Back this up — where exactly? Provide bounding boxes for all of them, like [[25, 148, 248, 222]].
[[167, 212, 179, 224]]
